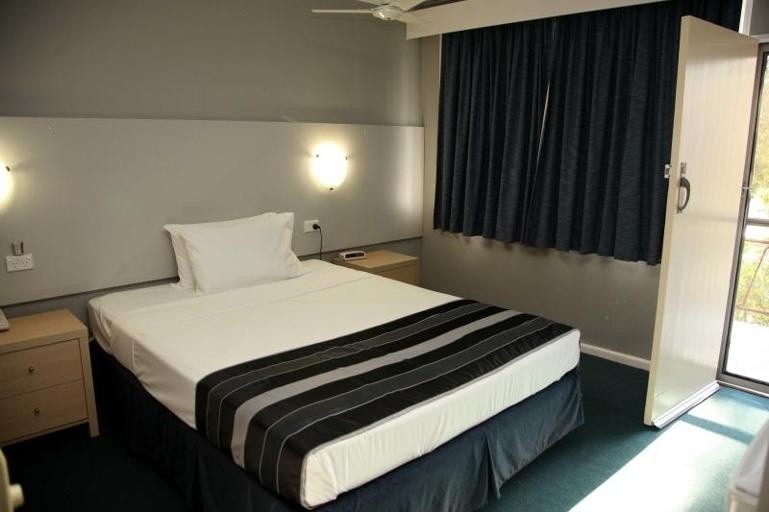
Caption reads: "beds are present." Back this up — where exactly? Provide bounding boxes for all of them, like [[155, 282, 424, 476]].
[[86, 259, 586, 512]]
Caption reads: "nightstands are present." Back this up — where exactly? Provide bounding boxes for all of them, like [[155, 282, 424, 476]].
[[333, 249, 420, 288]]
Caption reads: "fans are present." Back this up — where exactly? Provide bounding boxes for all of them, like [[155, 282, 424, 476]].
[[310, 1, 433, 28]]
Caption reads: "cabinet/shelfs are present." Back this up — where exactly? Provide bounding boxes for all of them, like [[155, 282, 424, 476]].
[[0, 308, 100, 447]]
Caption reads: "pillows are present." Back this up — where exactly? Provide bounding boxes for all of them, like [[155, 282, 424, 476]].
[[182, 212, 313, 296], [163, 212, 277, 291]]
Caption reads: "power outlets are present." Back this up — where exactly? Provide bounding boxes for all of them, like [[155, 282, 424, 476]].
[[6, 254, 35, 272], [304, 219, 320, 233]]
